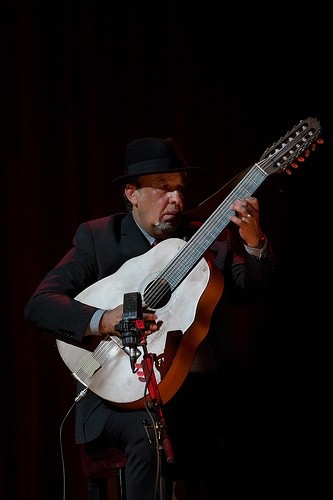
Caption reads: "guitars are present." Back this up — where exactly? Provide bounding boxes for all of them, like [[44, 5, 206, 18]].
[[56, 114, 324, 411]]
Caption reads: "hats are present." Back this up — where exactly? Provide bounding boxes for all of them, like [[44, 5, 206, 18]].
[[111, 138, 201, 181]]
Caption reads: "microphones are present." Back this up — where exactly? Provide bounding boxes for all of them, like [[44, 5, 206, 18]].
[[123, 292, 143, 371]]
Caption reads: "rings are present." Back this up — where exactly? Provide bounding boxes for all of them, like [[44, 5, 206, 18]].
[[242, 214, 251, 221]]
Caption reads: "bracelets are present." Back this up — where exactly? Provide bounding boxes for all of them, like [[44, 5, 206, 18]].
[[258, 240, 265, 249]]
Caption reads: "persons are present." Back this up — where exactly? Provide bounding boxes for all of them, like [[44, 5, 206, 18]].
[[26, 133, 280, 500]]
